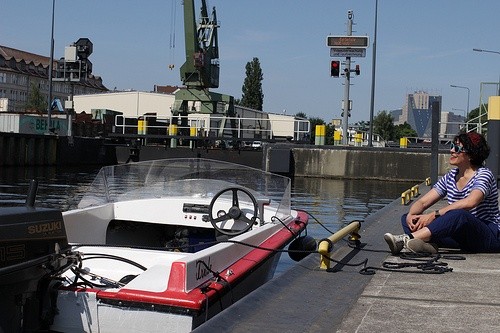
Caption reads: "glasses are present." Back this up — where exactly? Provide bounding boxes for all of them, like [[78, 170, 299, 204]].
[[450, 143, 469, 154]]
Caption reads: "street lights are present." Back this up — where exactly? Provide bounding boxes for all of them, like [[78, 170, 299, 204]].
[[449, 85, 470, 120], [452, 108, 466, 119], [473, 48, 500, 97]]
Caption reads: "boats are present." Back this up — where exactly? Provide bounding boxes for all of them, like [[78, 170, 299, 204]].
[[44, 157, 311, 332]]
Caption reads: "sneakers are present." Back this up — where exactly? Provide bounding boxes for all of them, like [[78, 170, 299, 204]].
[[384, 232, 410, 254], [406, 239, 439, 256]]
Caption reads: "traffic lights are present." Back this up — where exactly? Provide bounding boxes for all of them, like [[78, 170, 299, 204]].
[[331, 60, 340, 76]]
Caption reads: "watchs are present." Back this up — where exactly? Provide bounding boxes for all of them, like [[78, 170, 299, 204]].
[[434, 209, 440, 217]]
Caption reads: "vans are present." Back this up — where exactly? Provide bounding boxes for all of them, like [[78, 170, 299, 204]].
[[252, 140, 263, 150]]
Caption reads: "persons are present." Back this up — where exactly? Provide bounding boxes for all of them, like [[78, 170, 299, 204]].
[[384, 132, 500, 254]]
